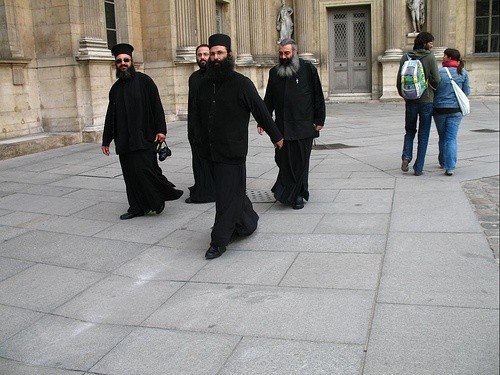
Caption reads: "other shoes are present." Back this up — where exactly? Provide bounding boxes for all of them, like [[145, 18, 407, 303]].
[[415, 171, 422, 175], [401, 160, 409, 171]]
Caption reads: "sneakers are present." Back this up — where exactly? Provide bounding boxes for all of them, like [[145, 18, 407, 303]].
[[445, 170, 453, 175]]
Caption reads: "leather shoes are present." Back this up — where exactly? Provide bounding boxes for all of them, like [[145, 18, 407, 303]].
[[120, 212, 144, 219], [204, 246, 226, 259], [293, 196, 304, 209], [155, 200, 164, 214]]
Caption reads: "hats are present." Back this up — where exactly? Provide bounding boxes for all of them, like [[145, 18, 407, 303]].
[[209, 34, 231, 49], [111, 43, 134, 54]]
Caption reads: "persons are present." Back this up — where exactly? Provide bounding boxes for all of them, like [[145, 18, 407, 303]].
[[200, 34, 283, 259], [101, 43, 183, 219], [276, 0, 295, 44], [408, 0, 425, 33], [397, 32, 441, 176], [433, 48, 470, 175], [186, 44, 217, 203], [257, 37, 326, 209]]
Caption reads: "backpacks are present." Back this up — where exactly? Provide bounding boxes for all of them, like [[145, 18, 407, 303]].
[[401, 60, 427, 99]]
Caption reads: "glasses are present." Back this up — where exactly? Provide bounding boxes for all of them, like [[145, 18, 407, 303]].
[[279, 50, 292, 55], [115, 59, 131, 63], [209, 51, 227, 55]]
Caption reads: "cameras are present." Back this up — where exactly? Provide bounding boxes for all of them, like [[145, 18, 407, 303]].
[[157, 146, 171, 161]]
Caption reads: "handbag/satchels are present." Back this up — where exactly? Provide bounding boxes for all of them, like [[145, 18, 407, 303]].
[[444, 67, 470, 116]]
